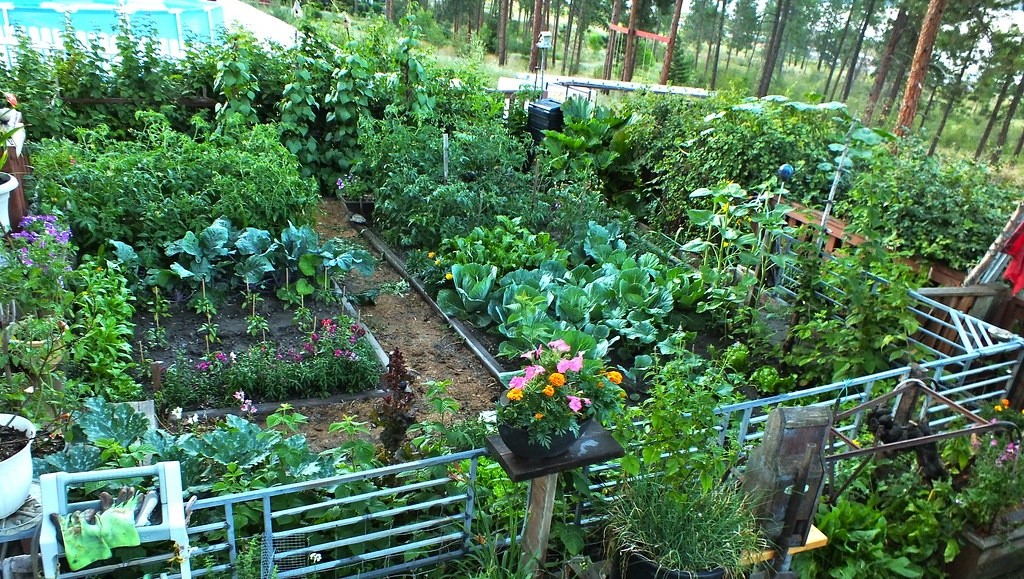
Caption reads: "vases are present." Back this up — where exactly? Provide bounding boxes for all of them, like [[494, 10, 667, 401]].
[[497, 403, 594, 460], [339, 196, 374, 222], [949, 499, 1024, 579]]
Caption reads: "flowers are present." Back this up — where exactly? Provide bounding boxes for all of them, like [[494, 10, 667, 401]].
[[337, 173, 366, 199], [485, 340, 627, 449], [955, 397, 1024, 540]]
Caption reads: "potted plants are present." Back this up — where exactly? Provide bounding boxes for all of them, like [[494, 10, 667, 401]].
[[0, 90, 32, 231], [0, 226, 115, 520], [589, 456, 788, 579]]
